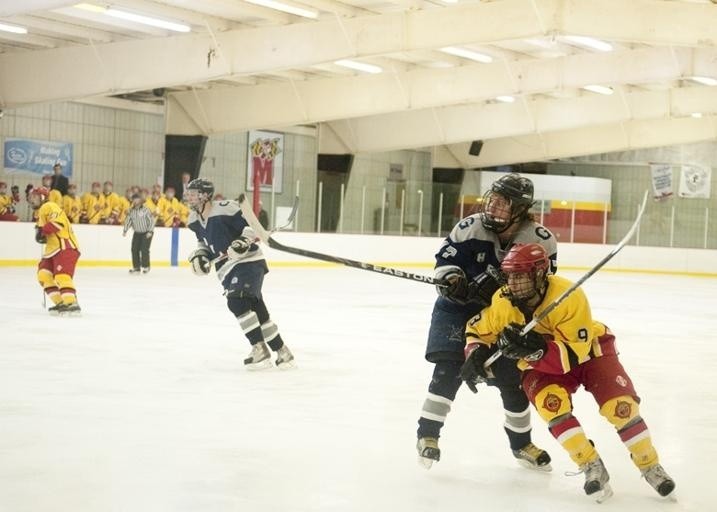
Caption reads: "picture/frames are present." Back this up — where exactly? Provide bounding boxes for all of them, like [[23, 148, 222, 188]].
[[245, 128, 286, 193]]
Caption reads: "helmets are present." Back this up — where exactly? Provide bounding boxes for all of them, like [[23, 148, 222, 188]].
[[68, 184, 77, 193], [504, 242, 548, 302], [29, 187, 48, 208], [92, 182, 101, 194], [104, 181, 113, 192], [130, 193, 145, 201], [42, 175, 52, 186], [481, 173, 535, 231], [127, 183, 176, 198], [185, 178, 214, 207], [0, 183, 8, 193]]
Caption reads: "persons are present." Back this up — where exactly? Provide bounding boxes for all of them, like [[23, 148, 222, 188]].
[[10, 185, 22, 213], [82, 181, 106, 224], [0, 181, 19, 222], [460, 242, 678, 504], [118, 188, 134, 225], [100, 181, 122, 224], [184, 177, 296, 372], [138, 189, 155, 212], [63, 184, 83, 223], [28, 185, 82, 317], [131, 186, 141, 197], [122, 193, 155, 274], [16, 184, 34, 222], [416, 172, 558, 472], [153, 186, 189, 227], [41, 175, 63, 210], [50, 164, 69, 197]]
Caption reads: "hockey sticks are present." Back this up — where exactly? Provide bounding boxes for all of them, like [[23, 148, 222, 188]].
[[204, 196, 300, 267], [238, 192, 453, 288], [484, 189, 650, 371], [41, 243, 46, 309]]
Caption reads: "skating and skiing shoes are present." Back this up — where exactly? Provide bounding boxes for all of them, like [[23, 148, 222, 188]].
[[581, 457, 613, 504], [418, 435, 440, 468], [48, 301, 80, 316], [140, 266, 151, 273], [276, 342, 295, 370], [511, 440, 553, 471], [643, 464, 679, 503], [129, 266, 142, 274], [244, 343, 273, 369]]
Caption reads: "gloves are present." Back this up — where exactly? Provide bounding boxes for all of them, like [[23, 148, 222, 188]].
[[500, 322, 548, 362], [467, 272, 502, 308], [228, 235, 252, 257], [461, 346, 492, 391], [190, 244, 213, 274], [37, 232, 47, 245], [434, 266, 468, 302]]
